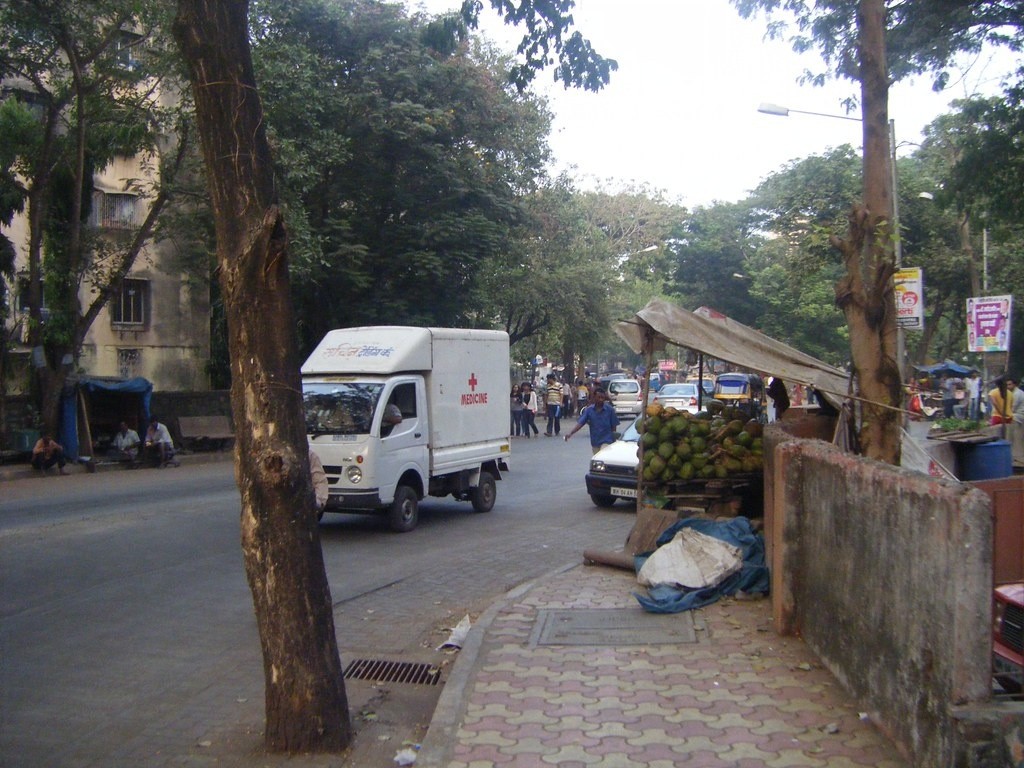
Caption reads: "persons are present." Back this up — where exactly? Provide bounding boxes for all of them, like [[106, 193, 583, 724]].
[[510, 367, 1024, 454], [308, 395, 402, 431], [106, 420, 140, 462], [31, 429, 71, 476], [143, 415, 174, 462], [308, 451, 329, 517]]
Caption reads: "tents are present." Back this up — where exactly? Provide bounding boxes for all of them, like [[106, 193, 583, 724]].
[[908, 360, 975, 379], [55, 373, 154, 457]]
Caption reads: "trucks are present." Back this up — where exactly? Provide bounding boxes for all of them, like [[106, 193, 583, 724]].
[[686, 376, 714, 393]]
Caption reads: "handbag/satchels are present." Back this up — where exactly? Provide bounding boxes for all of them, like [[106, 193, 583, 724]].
[[980, 401, 987, 414]]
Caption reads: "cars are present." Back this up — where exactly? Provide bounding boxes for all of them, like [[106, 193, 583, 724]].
[[585, 404, 708, 507], [652, 383, 708, 415]]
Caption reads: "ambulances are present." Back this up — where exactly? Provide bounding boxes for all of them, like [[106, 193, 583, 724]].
[[300, 326, 511, 532]]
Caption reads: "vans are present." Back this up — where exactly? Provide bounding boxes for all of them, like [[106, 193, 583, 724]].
[[713, 373, 767, 424], [649, 372, 660, 383], [607, 374, 627, 379], [605, 379, 644, 419]]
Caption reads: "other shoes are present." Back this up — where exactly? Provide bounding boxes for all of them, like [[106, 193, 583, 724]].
[[525, 435, 530, 439], [535, 433, 538, 438], [555, 432, 559, 436], [59, 469, 70, 475], [544, 432, 552, 437]]
